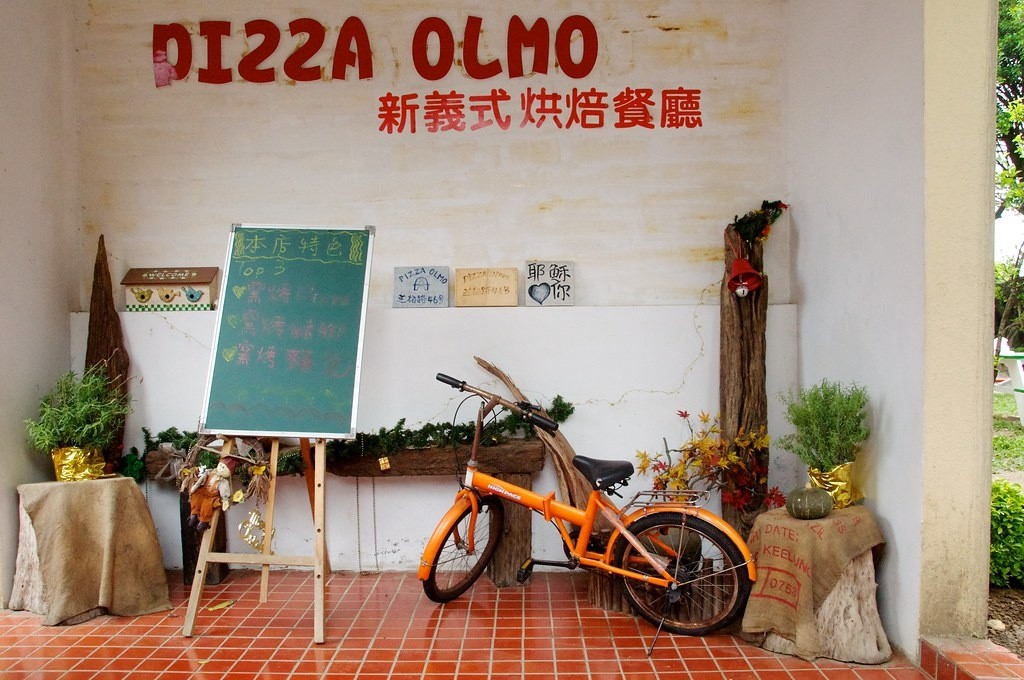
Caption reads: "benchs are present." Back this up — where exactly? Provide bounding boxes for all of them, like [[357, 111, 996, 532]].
[[143, 437, 545, 586]]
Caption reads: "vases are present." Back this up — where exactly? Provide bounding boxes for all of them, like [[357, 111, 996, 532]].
[[639, 528, 702, 581]]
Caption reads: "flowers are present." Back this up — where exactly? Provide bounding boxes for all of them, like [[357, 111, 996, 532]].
[[635, 409, 783, 505]]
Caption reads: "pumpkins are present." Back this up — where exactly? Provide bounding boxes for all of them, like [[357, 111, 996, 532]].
[[785, 482, 834, 519]]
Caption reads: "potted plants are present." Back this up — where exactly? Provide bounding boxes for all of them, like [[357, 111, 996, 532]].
[[775, 375, 871, 508], [23, 365, 135, 482]]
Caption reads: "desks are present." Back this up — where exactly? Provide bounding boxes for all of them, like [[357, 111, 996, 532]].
[[994, 353, 1024, 427], [739, 502, 893, 662], [7, 476, 173, 626]]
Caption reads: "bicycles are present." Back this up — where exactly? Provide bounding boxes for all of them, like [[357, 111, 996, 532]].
[[418, 372, 757, 656]]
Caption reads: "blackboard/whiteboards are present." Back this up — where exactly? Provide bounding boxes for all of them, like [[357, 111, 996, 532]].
[[197, 222, 378, 442]]
